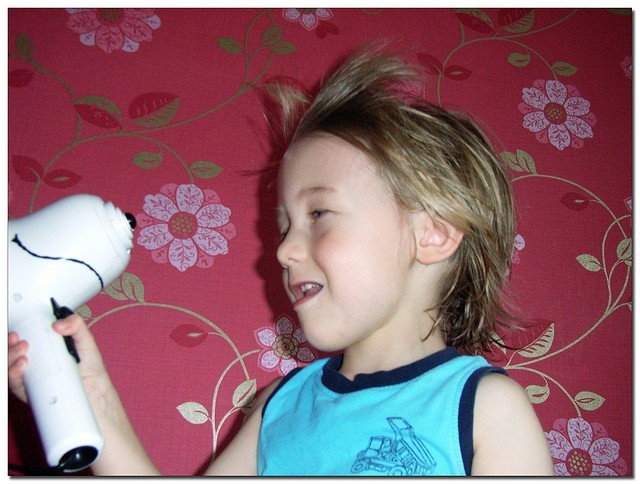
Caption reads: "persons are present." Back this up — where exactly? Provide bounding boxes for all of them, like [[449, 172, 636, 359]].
[[7, 52, 558, 477]]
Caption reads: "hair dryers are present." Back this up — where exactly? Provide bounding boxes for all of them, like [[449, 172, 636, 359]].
[[7, 193, 136, 474]]
